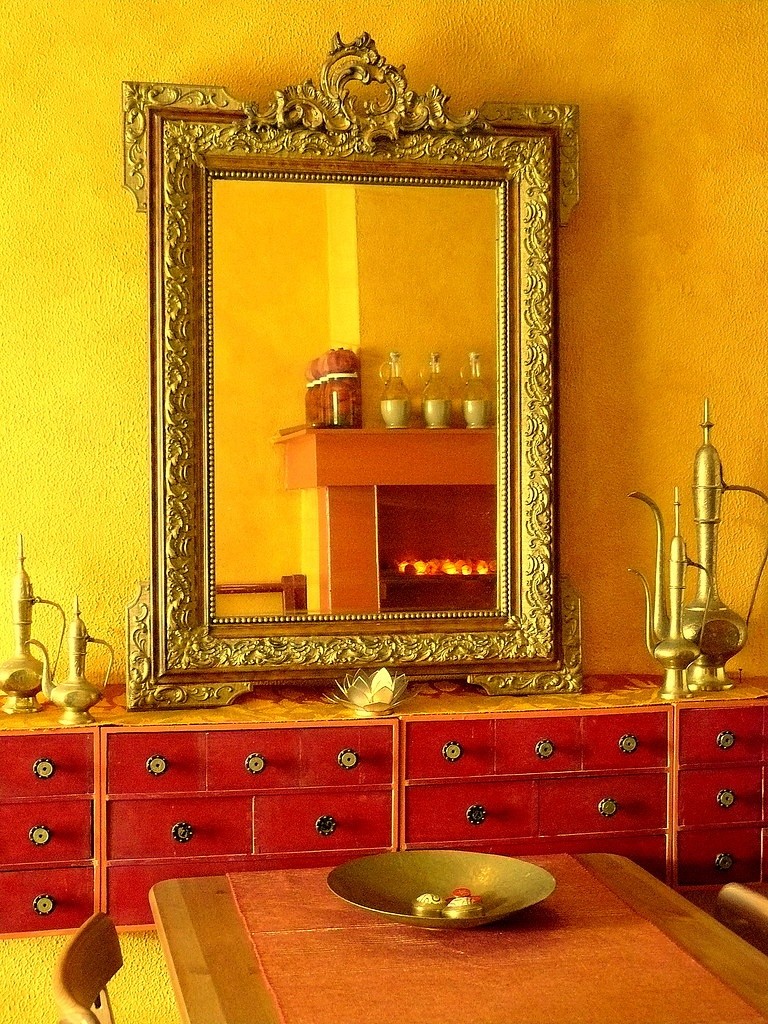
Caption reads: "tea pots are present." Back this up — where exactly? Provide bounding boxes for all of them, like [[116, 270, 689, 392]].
[[0, 534, 65, 713], [627, 487, 713, 700], [24, 595, 114, 725], [627, 397, 768, 692]]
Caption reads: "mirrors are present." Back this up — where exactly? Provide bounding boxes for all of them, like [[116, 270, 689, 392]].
[[122, 33, 583, 709]]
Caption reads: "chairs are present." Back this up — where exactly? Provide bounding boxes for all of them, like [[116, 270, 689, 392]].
[[714, 881, 768, 956], [50, 912, 126, 1024]]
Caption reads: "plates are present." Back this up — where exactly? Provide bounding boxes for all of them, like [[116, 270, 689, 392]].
[[324, 849, 555, 928]]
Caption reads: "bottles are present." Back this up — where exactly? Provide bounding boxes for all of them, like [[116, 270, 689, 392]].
[[459, 351, 491, 428], [419, 352, 453, 429], [306, 373, 360, 428], [379, 351, 411, 429]]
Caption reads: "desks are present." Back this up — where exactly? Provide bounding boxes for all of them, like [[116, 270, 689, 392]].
[[148, 852, 768, 1023]]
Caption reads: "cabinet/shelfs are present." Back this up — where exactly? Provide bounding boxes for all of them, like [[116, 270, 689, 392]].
[[0, 677, 767, 942]]
